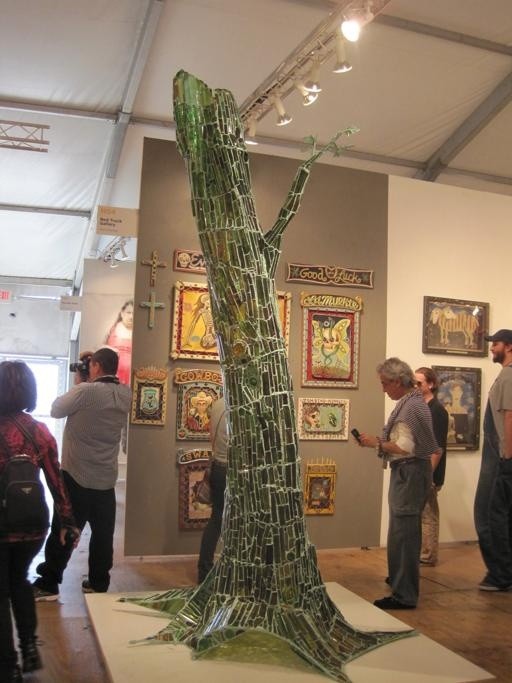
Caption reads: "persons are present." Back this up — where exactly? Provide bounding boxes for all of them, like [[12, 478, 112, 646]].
[[100, 294, 136, 456], [196, 393, 230, 589], [30, 345, 132, 603], [354, 354, 434, 610], [0, 356, 82, 680], [411, 365, 451, 568], [473, 327, 512, 592], [443, 381, 469, 442]]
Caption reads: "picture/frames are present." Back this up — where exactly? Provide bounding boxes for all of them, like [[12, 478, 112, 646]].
[[297, 398, 353, 442], [173, 368, 224, 444], [298, 291, 363, 390], [170, 278, 292, 364], [304, 462, 336, 516], [175, 446, 216, 531], [431, 366, 482, 452], [422, 295, 490, 359], [129, 363, 168, 427]]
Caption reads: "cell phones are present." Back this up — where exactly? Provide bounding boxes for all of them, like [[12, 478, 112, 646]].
[[58, 527, 78, 562], [351, 428, 362, 442]]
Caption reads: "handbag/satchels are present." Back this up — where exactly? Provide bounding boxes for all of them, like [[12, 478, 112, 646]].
[[194, 469, 212, 504]]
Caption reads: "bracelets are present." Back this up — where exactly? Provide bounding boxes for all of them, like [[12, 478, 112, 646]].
[[376, 437, 383, 457]]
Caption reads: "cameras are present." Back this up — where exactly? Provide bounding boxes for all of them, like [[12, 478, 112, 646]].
[[69, 356, 90, 382]]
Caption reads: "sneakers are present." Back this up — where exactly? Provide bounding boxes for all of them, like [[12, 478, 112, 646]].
[[479, 581, 511, 591], [8, 665, 22, 682], [82, 580, 95, 592], [29, 585, 60, 603], [374, 597, 415, 610], [21, 641, 42, 672]]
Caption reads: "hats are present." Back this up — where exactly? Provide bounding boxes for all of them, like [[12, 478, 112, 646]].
[[191, 393, 212, 407], [485, 329, 512, 343]]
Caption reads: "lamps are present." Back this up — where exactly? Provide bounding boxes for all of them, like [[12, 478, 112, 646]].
[[244, 1, 381, 147]]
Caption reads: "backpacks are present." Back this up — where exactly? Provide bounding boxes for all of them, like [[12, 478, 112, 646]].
[[1, 419, 49, 555]]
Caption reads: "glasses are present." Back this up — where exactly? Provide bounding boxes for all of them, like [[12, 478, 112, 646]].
[[417, 381, 424, 387]]
